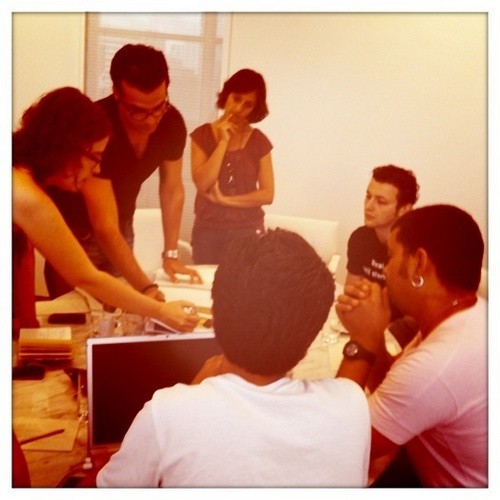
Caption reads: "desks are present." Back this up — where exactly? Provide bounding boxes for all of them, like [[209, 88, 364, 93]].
[[12, 264, 401, 488]]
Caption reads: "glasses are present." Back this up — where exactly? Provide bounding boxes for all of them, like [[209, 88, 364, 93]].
[[119, 90, 169, 120], [79, 151, 105, 170]]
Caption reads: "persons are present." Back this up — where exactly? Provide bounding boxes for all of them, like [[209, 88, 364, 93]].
[[346, 164, 419, 394], [12, 87, 201, 335], [96, 227, 373, 488], [43, 44, 203, 300], [190, 68, 275, 265], [335, 205, 488, 488]]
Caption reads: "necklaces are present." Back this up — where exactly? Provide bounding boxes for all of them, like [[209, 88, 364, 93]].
[[422, 296, 476, 335]]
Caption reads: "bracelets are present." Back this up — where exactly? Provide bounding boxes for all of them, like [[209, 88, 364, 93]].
[[162, 248, 179, 259], [140, 282, 159, 294]]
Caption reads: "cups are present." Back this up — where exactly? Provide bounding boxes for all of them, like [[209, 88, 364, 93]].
[[95, 312, 145, 338]]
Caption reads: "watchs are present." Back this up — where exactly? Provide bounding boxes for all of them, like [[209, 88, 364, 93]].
[[343, 339, 377, 365]]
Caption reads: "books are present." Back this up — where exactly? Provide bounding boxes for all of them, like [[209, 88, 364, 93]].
[[18, 327, 74, 362], [152, 263, 219, 314]]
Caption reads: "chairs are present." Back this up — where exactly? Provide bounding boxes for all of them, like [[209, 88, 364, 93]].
[[263, 215, 337, 264], [132, 209, 164, 270]]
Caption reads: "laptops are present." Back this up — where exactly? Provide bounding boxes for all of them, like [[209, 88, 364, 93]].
[[85, 331, 225, 478]]
